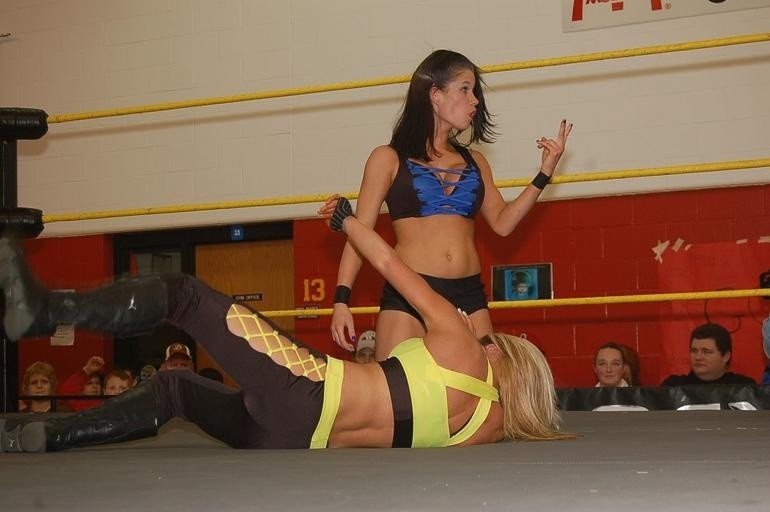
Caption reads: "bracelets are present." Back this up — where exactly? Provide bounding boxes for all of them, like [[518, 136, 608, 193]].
[[331, 284, 353, 306], [530, 170, 552, 192]]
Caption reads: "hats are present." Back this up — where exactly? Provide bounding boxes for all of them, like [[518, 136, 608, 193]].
[[355, 330, 376, 352], [165, 343, 192, 360]]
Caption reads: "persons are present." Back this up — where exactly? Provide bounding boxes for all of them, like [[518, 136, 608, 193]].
[[15, 345, 224, 417], [355, 330, 377, 363], [327, 45, 575, 365], [588, 344, 632, 387], [0, 192, 581, 454], [655, 322, 770, 412]]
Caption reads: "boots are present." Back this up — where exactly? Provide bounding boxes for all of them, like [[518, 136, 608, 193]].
[[0, 380, 159, 452], [0, 234, 170, 342]]
